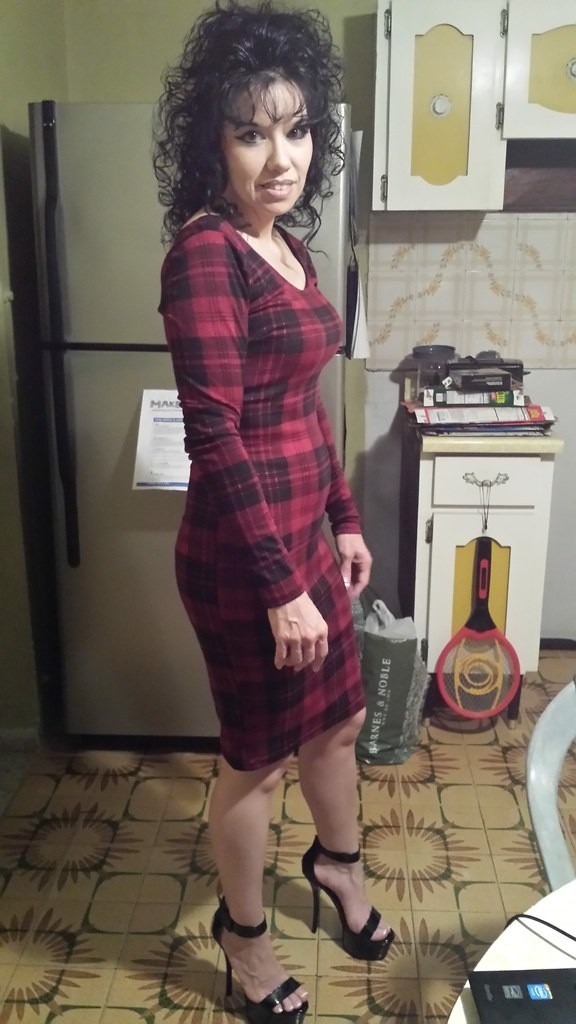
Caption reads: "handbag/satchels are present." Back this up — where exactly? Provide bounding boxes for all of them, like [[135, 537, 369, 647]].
[[363, 630, 429, 766]]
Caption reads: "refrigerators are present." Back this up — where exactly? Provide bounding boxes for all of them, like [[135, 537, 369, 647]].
[[28, 100, 346, 751]]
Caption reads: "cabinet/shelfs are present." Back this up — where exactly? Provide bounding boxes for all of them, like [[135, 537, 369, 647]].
[[502, 1, 576, 139], [396, 427, 565, 731], [372, 0, 511, 213]]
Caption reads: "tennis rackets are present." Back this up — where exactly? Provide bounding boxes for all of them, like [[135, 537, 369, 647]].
[[435, 537, 521, 719]]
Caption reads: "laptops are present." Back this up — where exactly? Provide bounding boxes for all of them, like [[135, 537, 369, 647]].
[[467, 968, 576, 1024]]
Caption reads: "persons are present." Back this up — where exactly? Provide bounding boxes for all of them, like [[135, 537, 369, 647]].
[[150, 1, 395, 1024]]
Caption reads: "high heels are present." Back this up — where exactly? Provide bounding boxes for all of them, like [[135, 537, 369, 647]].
[[212, 896, 310, 1024], [300, 835, 395, 963]]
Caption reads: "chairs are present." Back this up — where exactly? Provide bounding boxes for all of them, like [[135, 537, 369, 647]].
[[525, 674, 575, 890]]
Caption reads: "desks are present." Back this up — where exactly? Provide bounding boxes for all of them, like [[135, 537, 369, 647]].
[[446, 880, 576, 1024]]
